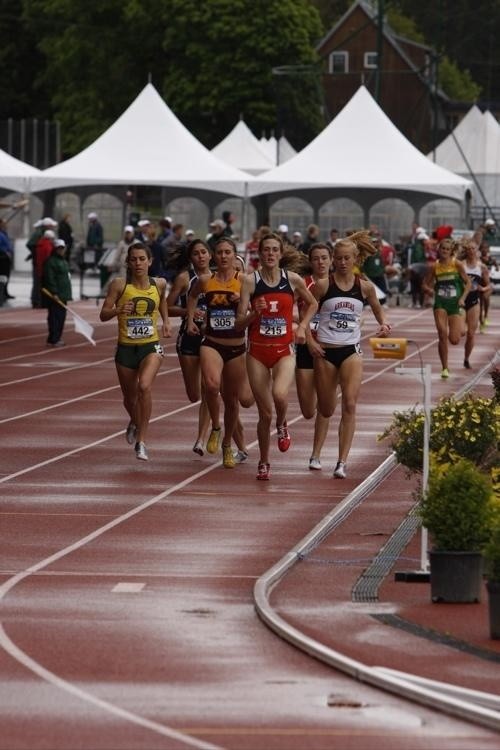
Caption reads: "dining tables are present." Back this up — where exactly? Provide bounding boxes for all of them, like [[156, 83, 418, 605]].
[[412, 460, 500, 604], [480, 510, 500, 640]]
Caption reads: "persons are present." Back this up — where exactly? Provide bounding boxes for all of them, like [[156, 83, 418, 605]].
[[473, 232, 484, 246], [107, 225, 144, 282], [56, 213, 76, 255], [0, 212, 16, 308], [162, 224, 185, 250], [220, 211, 238, 250], [233, 236, 319, 481], [400, 262, 428, 308], [368, 224, 379, 236], [159, 219, 171, 243], [302, 241, 391, 478], [27, 216, 59, 249], [362, 236, 390, 312], [33, 220, 43, 232], [138, 218, 155, 253], [278, 224, 292, 244], [328, 229, 343, 246], [181, 228, 195, 250], [244, 232, 260, 272], [187, 238, 252, 469], [292, 232, 304, 248], [426, 239, 473, 378], [160, 241, 218, 457], [422, 231, 441, 307], [459, 241, 492, 370], [411, 220, 428, 242], [41, 240, 74, 348], [258, 226, 271, 237], [345, 229, 356, 237], [100, 243, 171, 463], [205, 218, 223, 248], [32, 231, 55, 306], [380, 238, 407, 276], [233, 256, 244, 279], [298, 224, 318, 254], [479, 243, 493, 335], [86, 212, 104, 251]]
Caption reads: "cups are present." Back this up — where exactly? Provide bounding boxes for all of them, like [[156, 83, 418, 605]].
[[372, 240, 380, 244]]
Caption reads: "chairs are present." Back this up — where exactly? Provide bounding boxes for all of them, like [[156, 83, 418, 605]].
[[463, 360, 473, 369], [416, 305, 424, 310], [480, 324, 485, 336], [276, 418, 291, 452], [485, 317, 489, 328], [126, 423, 137, 445], [408, 301, 415, 309], [221, 442, 236, 469], [207, 428, 221, 455], [235, 448, 249, 464], [56, 338, 66, 345], [442, 368, 448, 378], [134, 441, 148, 463], [332, 461, 346, 478], [46, 341, 61, 349], [382, 304, 389, 309], [307, 455, 322, 471], [255, 460, 272, 481]]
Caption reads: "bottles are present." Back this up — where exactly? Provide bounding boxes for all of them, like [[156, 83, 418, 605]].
[[210, 219, 226, 230], [205, 233, 213, 240], [416, 227, 425, 235], [43, 217, 57, 227], [165, 217, 173, 224], [88, 213, 99, 221], [293, 232, 302, 238], [43, 230, 55, 239], [417, 233, 428, 241], [278, 224, 289, 233], [137, 220, 151, 227], [435, 225, 453, 242], [124, 225, 135, 233], [54, 239, 65, 249], [33, 219, 43, 228], [484, 219, 496, 227], [185, 229, 194, 236]]
